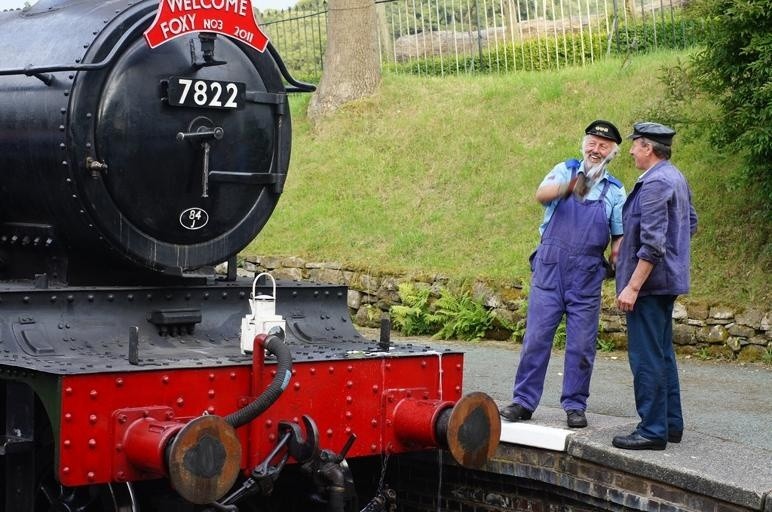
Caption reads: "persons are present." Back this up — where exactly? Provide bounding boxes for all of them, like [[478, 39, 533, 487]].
[[611, 122, 708, 450], [499, 119, 628, 430]]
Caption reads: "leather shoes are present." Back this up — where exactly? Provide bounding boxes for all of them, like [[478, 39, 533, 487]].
[[500, 402, 533, 422], [612, 430, 681, 450], [567, 409, 588, 427]]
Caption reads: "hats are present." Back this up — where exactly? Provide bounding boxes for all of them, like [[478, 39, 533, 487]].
[[586, 120, 622, 145], [626, 122, 677, 146]]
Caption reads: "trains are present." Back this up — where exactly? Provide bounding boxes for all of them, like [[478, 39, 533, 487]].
[[0, 0, 503, 509]]
